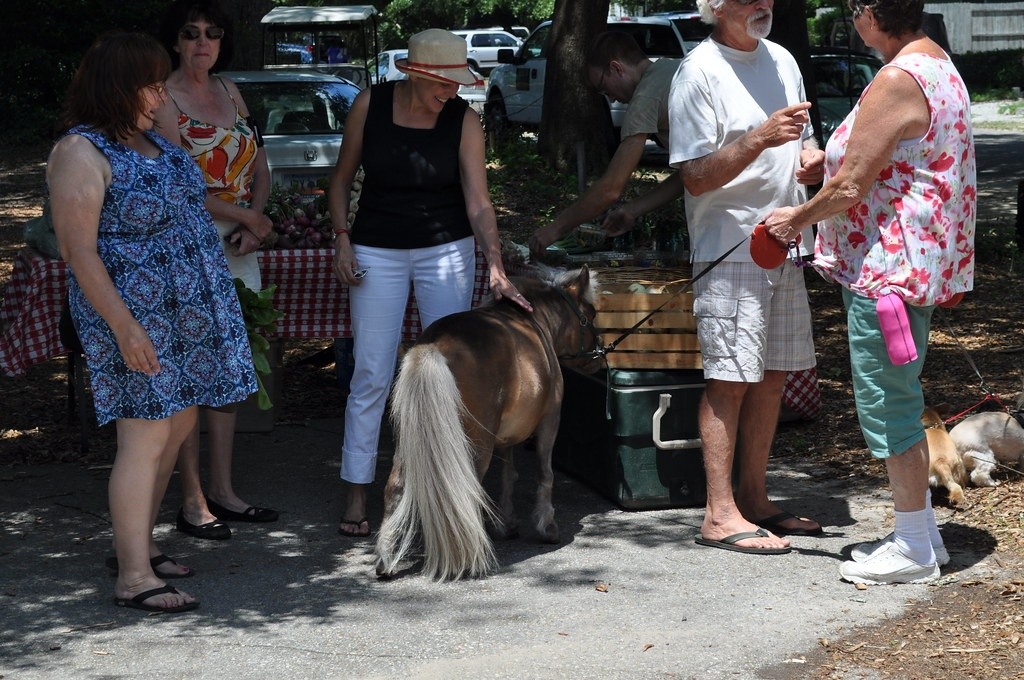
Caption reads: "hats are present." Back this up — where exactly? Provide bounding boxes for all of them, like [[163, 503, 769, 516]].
[[395, 28, 478, 86]]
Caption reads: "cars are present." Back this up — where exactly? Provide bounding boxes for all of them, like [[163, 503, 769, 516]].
[[647, 8, 717, 54], [366, 25, 542, 121], [809, 44, 885, 149], [211, 71, 365, 206]]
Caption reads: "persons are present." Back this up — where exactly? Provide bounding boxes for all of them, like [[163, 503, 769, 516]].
[[667, 0, 824, 555], [767, 0, 977, 586], [164, 0, 273, 541], [47, 28, 259, 613], [528, 31, 686, 262], [320, 28, 534, 538], [322, 39, 356, 76]]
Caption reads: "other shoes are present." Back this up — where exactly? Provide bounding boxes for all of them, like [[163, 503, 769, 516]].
[[204, 495, 279, 522], [176, 505, 231, 540]]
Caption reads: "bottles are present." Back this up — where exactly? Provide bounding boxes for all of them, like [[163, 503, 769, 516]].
[[875, 287, 919, 367]]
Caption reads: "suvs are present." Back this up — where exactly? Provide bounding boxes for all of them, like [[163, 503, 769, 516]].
[[482, 15, 688, 159]]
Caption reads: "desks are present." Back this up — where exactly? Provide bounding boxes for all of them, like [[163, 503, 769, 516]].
[[1, 240, 494, 459]]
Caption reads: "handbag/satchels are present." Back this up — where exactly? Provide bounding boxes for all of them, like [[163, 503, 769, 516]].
[[246, 115, 264, 147]]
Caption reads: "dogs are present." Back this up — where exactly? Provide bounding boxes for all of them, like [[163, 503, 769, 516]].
[[920, 408, 968, 509], [949, 411, 1024, 487]]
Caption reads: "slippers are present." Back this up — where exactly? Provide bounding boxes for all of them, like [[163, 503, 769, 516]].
[[114, 585, 199, 612], [106, 553, 192, 578], [695, 525, 792, 554], [338, 512, 371, 537], [741, 511, 822, 535], [851, 530, 950, 567], [839, 542, 941, 586]]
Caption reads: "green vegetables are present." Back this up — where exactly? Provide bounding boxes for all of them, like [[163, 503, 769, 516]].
[[233, 277, 285, 411]]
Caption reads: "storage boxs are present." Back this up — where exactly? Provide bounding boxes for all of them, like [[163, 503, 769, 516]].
[[526, 368, 740, 510]]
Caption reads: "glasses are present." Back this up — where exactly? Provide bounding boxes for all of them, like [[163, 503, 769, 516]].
[[143, 83, 167, 94], [733, 0, 759, 5], [595, 57, 619, 97], [177, 25, 224, 40]]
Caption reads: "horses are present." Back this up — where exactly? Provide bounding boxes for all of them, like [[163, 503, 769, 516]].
[[376, 262, 612, 580]]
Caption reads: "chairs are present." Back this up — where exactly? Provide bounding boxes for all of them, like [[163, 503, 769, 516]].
[[284, 111, 322, 130]]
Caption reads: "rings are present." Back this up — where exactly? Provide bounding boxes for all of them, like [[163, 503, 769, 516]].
[[516, 293, 522, 298]]
